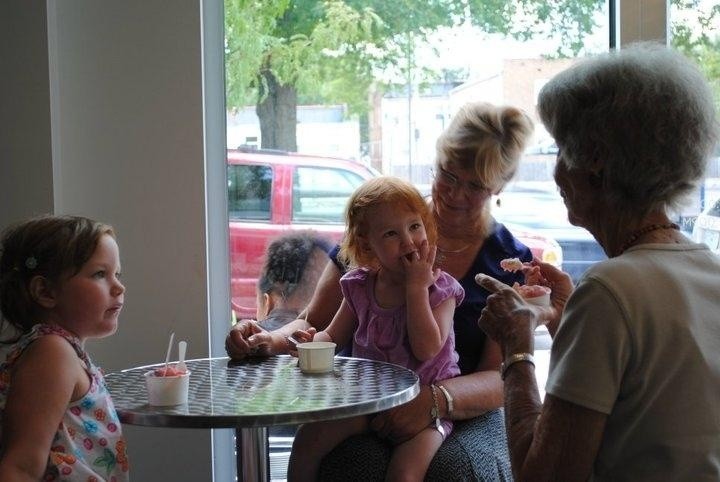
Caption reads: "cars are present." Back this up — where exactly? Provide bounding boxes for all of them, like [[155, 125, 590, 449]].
[[421, 183, 613, 293]]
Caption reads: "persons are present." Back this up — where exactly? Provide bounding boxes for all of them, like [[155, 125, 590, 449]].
[[472, 39, 720, 482], [1, 209, 131, 481], [242, 225, 335, 436], [222, 101, 535, 482], [286, 175, 460, 481]]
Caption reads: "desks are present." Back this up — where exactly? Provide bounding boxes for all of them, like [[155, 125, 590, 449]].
[[102, 354, 420, 482]]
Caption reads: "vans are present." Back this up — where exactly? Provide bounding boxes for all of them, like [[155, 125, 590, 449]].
[[227, 149, 377, 320]]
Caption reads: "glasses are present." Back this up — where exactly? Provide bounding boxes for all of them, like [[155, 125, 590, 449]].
[[439, 168, 489, 194]]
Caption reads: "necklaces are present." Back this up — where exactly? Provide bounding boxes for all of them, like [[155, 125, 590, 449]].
[[612, 220, 680, 256]]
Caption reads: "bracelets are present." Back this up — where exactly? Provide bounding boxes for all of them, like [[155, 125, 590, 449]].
[[431, 382, 444, 435], [499, 351, 535, 377], [437, 381, 453, 419]]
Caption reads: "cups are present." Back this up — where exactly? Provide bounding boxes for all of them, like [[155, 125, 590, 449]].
[[296, 342, 336, 373], [143, 369, 190, 406], [513, 286, 551, 307]]
